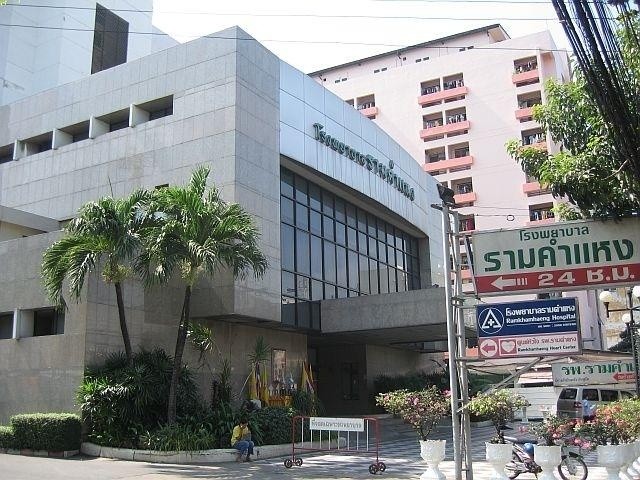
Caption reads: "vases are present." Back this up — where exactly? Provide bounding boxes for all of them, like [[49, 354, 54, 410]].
[[485, 440, 513, 480], [532, 444, 562, 480], [596, 440, 640, 479], [420, 438, 446, 480]]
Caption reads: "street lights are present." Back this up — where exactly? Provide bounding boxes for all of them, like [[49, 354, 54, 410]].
[[622, 312, 639, 335], [599, 284, 640, 399], [437, 183, 472, 480]]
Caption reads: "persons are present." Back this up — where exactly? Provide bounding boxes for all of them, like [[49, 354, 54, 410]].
[[230, 419, 255, 462], [582, 395, 591, 423]]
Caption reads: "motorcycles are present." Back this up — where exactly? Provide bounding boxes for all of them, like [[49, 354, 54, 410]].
[[500, 429, 589, 480]]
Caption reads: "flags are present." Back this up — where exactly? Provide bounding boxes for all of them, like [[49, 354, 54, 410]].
[[249, 359, 318, 406]]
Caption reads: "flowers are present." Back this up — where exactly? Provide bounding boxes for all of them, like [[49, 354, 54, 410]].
[[468, 388, 531, 444], [577, 397, 640, 442], [374, 380, 451, 441], [519, 412, 586, 447]]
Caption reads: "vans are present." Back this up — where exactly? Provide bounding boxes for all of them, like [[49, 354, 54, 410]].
[[556, 386, 635, 422]]
